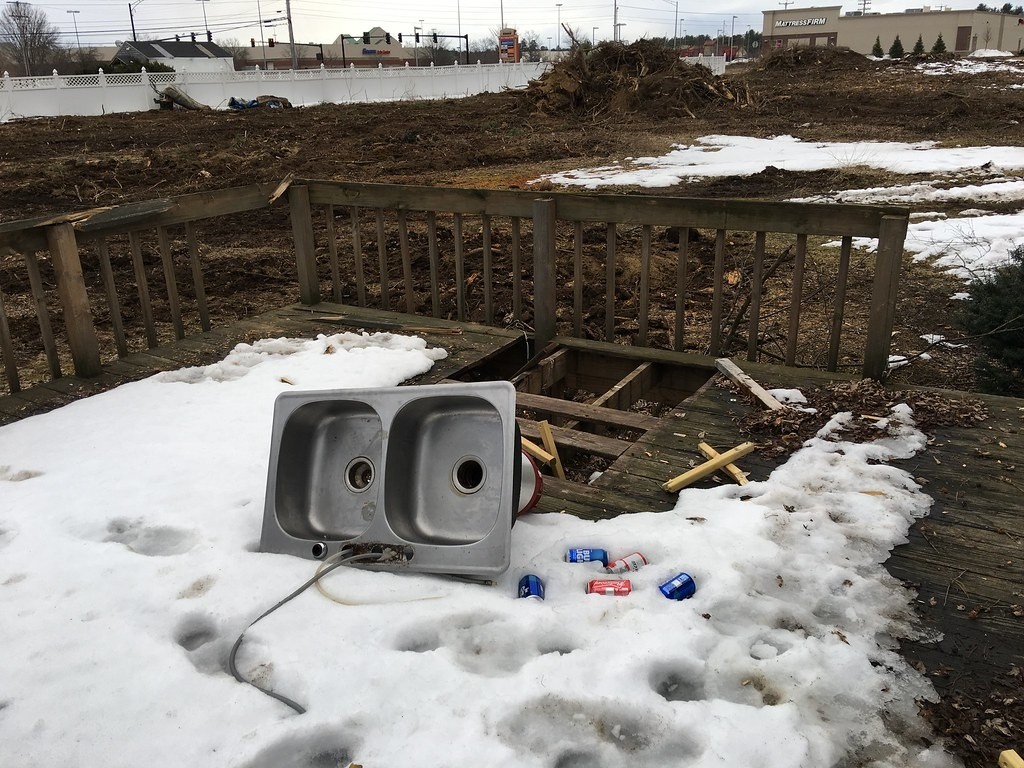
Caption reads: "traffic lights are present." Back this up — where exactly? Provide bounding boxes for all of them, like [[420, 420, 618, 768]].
[[432, 33, 437, 43], [176, 34, 180, 42], [268, 38, 274, 47], [207, 31, 212, 43], [398, 33, 402, 42], [415, 32, 419, 43], [363, 32, 370, 44], [385, 33, 391, 45], [251, 38, 255, 48], [190, 32, 196, 42]]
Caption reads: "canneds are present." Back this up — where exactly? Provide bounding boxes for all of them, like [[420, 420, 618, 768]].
[[518, 574, 544, 601], [567, 548, 609, 568], [587, 579, 632, 596], [607, 552, 647, 575], [659, 572, 695, 601]]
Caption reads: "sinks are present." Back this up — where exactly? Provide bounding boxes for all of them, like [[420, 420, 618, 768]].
[[256, 386, 382, 573], [381, 381, 517, 580]]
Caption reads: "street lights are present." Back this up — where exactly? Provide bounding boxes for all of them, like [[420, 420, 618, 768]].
[[547, 3, 755, 62]]
